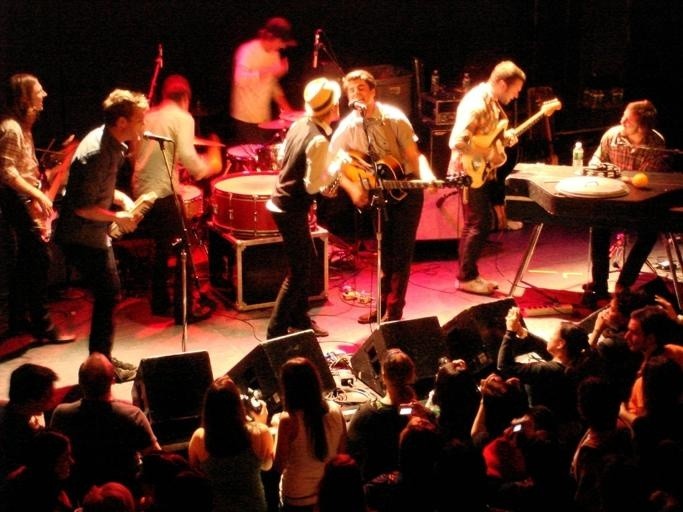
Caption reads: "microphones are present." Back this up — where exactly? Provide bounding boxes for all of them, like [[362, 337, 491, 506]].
[[141, 131, 173, 142], [346, 99, 368, 113]]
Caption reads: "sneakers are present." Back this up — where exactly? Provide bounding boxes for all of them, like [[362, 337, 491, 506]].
[[476, 276, 499, 289], [455, 279, 493, 295], [289, 320, 328, 337], [108, 356, 138, 371], [112, 368, 137, 382]]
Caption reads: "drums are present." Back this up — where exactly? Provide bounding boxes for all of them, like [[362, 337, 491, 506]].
[[226, 144, 265, 172], [210, 170, 318, 238], [178, 183, 204, 220]]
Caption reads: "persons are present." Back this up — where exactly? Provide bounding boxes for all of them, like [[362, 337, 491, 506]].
[[232, 15, 295, 145], [130, 73, 214, 326], [55, 87, 150, 385], [0, 73, 75, 345], [1, 284, 682, 511], [328, 68, 424, 325], [266, 78, 342, 341], [447, 61, 527, 296], [581, 101, 666, 296]]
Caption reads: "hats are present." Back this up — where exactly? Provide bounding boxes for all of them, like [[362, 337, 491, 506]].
[[266, 17, 296, 46], [303, 77, 341, 117], [603, 309, 623, 329]]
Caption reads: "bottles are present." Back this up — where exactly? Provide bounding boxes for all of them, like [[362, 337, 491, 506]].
[[431, 70, 439, 92], [572, 141, 585, 175], [462, 72, 470, 88]]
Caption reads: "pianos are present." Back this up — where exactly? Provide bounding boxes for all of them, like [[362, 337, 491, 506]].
[[504, 163, 683, 311]]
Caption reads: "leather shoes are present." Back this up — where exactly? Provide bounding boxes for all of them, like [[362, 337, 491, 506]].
[[177, 305, 211, 325], [34, 326, 76, 343], [357, 310, 385, 323], [614, 284, 629, 295], [380, 312, 403, 322], [151, 304, 173, 316], [582, 281, 608, 294]]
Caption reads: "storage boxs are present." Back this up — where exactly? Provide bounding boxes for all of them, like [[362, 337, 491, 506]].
[[206, 221, 329, 312], [418, 93, 461, 127], [412, 129, 521, 180]]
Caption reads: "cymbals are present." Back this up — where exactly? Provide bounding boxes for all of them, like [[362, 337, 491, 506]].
[[193, 137, 226, 147], [258, 120, 294, 130]]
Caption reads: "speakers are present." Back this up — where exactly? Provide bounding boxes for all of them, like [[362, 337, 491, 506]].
[[350, 316, 446, 395], [131, 350, 212, 446], [441, 296, 527, 359], [226, 327, 336, 415]]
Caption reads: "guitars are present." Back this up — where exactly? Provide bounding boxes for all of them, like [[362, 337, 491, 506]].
[[458, 98, 561, 190], [344, 150, 472, 213], [22, 140, 76, 244]]
